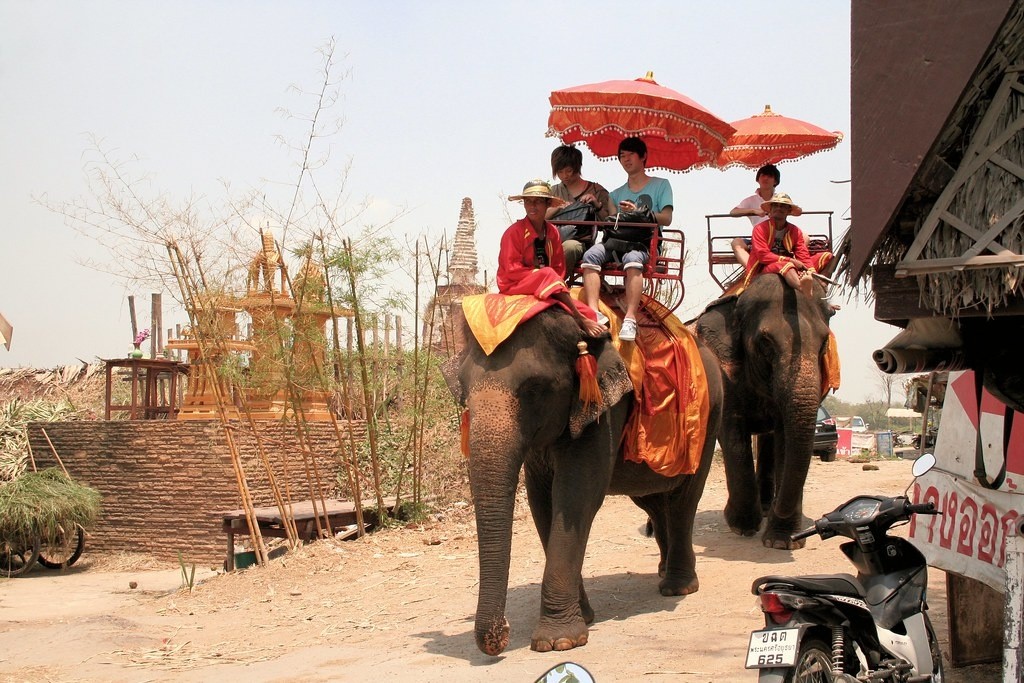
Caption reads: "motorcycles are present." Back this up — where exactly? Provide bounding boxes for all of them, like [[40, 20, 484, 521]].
[[745, 453, 946, 683]]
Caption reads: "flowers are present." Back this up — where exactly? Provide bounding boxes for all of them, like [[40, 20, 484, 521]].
[[132, 328, 152, 346]]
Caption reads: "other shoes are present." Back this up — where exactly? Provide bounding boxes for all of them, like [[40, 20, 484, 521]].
[[595, 311, 609, 326], [619, 318, 637, 341]]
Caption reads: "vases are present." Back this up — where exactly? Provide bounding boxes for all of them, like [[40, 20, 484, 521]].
[[132, 342, 143, 359]]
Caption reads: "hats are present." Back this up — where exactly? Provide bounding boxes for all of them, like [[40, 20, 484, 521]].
[[508, 178, 565, 207], [761, 192, 802, 217]]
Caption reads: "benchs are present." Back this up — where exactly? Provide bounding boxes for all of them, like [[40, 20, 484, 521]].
[[517, 219, 686, 328], [705, 210, 834, 290]]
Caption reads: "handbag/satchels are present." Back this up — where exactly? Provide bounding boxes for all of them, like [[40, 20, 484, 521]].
[[603, 206, 662, 254], [547, 201, 596, 243]]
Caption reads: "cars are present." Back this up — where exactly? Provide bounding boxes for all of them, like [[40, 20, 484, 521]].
[[852, 416, 870, 433], [810, 405, 839, 463]]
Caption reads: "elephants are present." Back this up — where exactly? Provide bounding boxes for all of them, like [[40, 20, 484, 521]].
[[437, 282, 724, 656], [683, 270, 835, 549]]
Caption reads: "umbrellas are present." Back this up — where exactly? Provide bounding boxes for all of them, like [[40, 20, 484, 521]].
[[708, 104, 844, 173], [544, 70, 738, 174]]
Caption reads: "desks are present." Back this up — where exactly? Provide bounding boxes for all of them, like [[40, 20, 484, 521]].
[[102, 357, 192, 420], [205, 494, 445, 577]]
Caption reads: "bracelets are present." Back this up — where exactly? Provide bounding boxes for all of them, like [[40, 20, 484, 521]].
[[594, 201, 603, 212]]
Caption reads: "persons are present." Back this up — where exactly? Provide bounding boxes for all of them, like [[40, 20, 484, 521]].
[[544, 145, 610, 282], [743, 192, 841, 310], [496, 179, 608, 337], [581, 137, 674, 340], [730, 165, 810, 270]]
